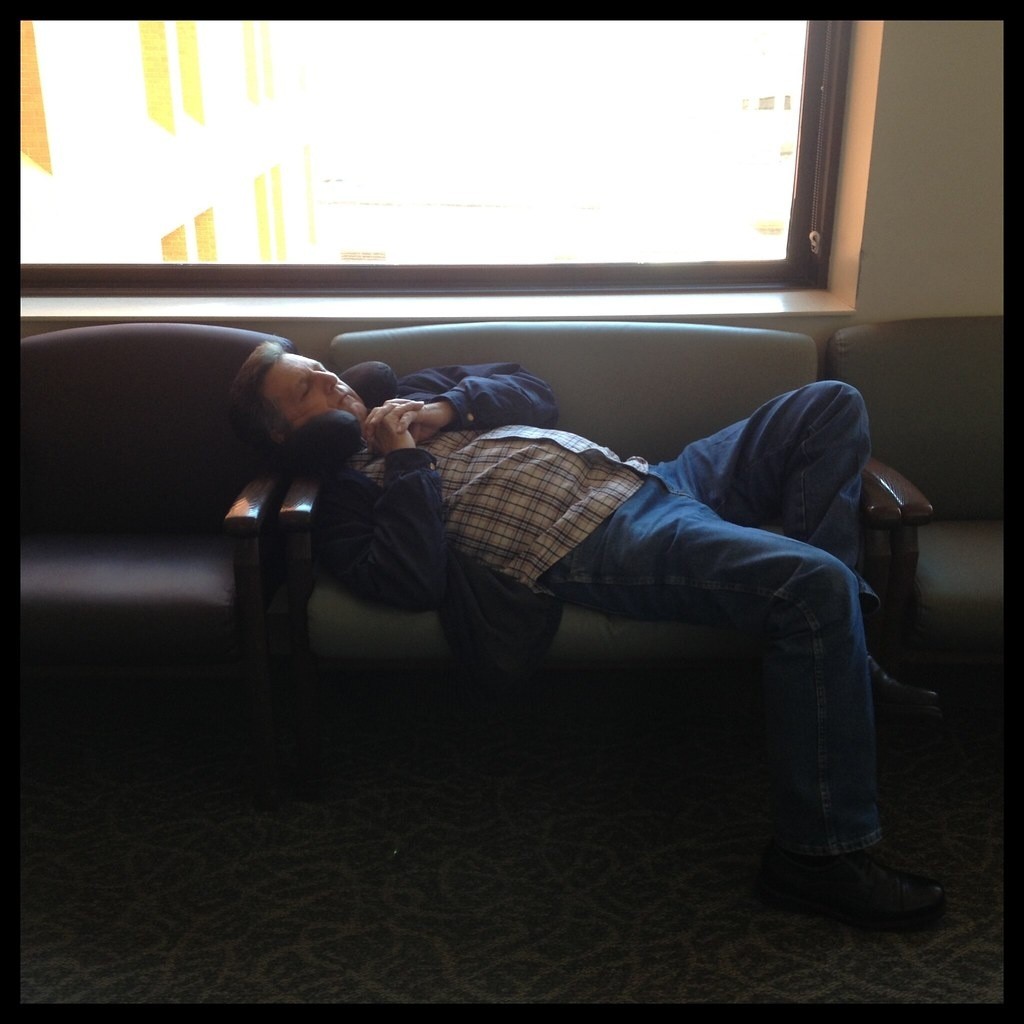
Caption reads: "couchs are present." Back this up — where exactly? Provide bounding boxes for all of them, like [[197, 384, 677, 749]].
[[21, 315, 1004, 809]]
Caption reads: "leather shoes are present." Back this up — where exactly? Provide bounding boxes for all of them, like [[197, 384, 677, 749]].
[[752, 838, 947, 929], [866, 649, 943, 721]]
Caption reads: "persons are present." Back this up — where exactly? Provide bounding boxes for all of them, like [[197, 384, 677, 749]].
[[233, 343, 947, 919]]
